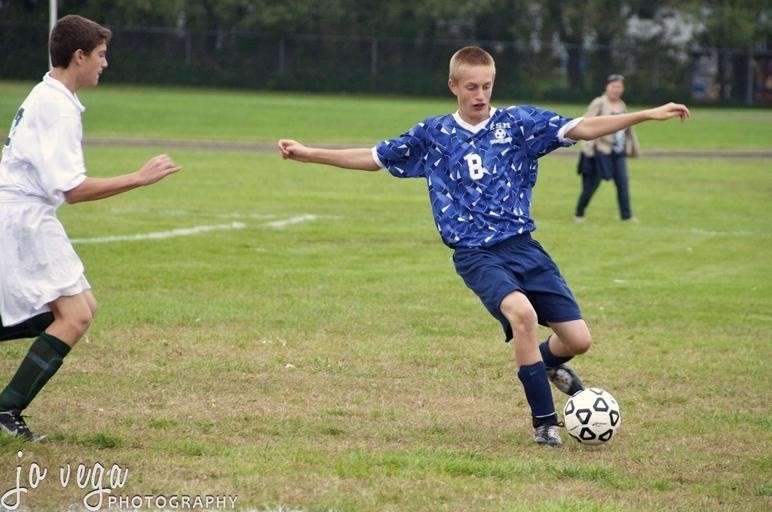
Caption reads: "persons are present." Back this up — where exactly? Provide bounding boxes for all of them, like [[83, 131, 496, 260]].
[[277, 47, 693, 446], [575, 70, 640, 222], [1, 15, 184, 444]]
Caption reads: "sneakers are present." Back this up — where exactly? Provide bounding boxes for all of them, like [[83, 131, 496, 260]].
[[544, 361, 585, 396], [533, 417, 564, 446], [0, 409, 38, 443]]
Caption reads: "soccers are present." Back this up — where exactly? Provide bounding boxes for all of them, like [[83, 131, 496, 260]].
[[564, 388, 620, 446]]
